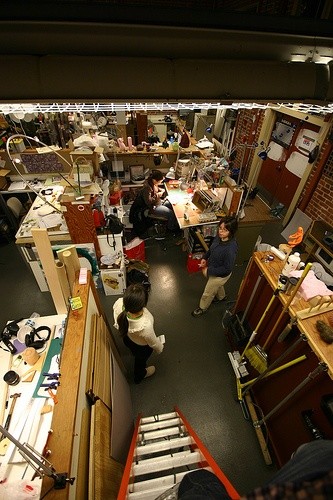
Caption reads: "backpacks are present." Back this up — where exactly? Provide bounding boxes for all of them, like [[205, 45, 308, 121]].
[[106, 214, 124, 234]]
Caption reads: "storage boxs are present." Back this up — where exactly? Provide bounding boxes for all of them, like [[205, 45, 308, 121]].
[[187, 227, 214, 253], [0, 170, 11, 189]]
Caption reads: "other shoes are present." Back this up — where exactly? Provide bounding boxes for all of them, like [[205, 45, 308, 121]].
[[144, 366, 156, 377], [192, 306, 208, 317], [213, 295, 229, 303]]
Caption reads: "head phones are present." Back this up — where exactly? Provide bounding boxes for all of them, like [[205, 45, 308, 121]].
[[25, 326, 51, 349], [0, 317, 23, 340]]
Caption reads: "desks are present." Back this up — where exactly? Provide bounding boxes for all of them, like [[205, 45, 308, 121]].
[[0, 110, 333, 500]]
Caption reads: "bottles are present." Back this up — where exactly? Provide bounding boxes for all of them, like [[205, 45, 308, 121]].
[[25, 312, 40, 327], [281, 252, 301, 277]]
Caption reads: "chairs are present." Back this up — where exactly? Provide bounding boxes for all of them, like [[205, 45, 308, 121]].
[[138, 190, 168, 237], [7, 197, 26, 227]]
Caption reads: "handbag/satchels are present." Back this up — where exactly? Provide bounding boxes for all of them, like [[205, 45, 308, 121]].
[[123, 237, 145, 262]]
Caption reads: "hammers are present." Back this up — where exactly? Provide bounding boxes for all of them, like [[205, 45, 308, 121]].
[[0, 393, 22, 441]]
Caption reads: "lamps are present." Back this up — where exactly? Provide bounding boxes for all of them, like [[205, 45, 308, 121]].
[[166, 167, 175, 179], [201, 117, 213, 133], [217, 138, 271, 185]]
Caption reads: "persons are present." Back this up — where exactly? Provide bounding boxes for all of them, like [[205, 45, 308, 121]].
[[141, 170, 182, 237], [192, 217, 238, 319], [113, 283, 164, 380], [177, 440, 333, 500]]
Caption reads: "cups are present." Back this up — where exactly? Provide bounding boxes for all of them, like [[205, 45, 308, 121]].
[[277, 275, 289, 292], [3, 371, 20, 385], [284, 276, 299, 295]]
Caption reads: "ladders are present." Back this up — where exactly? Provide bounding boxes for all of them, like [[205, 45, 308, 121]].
[[116, 404, 243, 500]]
